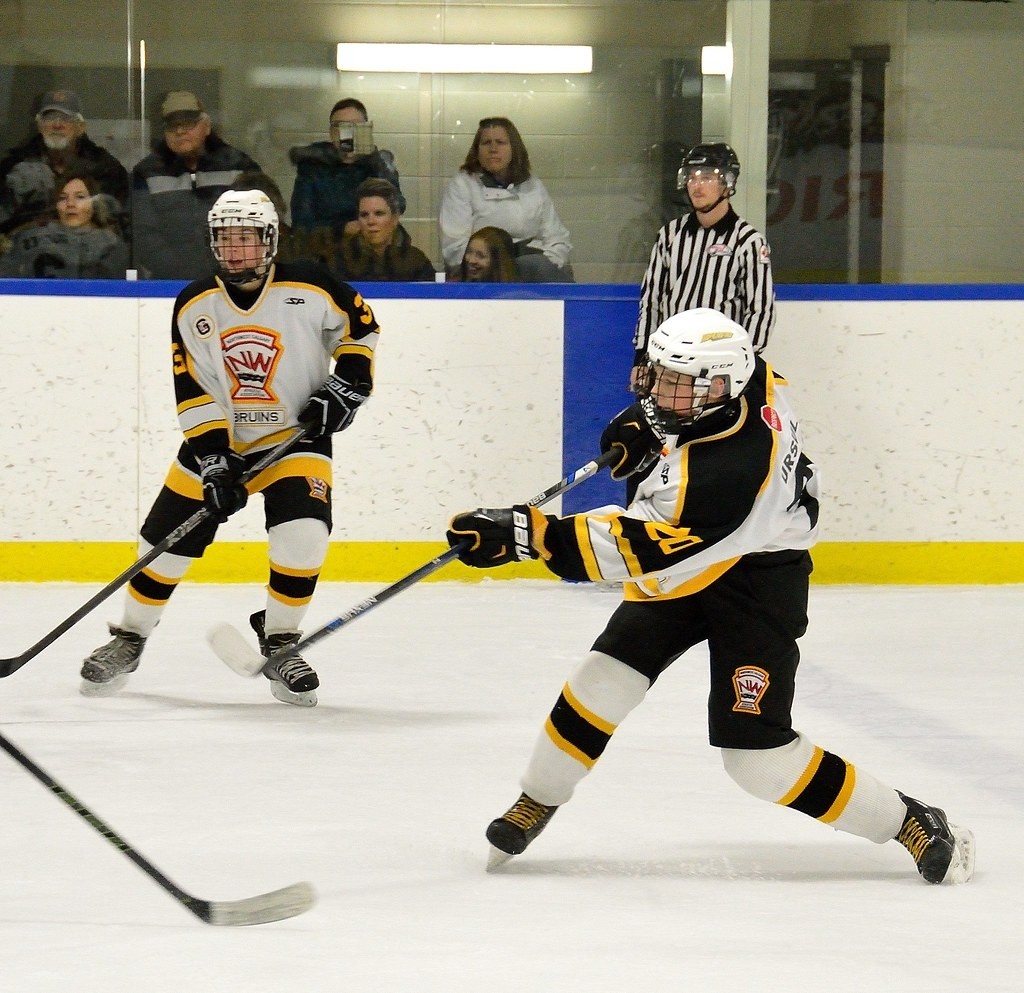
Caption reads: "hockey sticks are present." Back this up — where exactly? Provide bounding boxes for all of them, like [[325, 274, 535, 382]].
[[1, 430, 307, 681], [1, 733, 316, 925], [205, 449, 623, 676]]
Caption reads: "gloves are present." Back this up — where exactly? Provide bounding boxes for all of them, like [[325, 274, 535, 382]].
[[297, 365, 374, 440], [445, 506, 547, 570], [197, 446, 249, 525], [600, 401, 666, 484]]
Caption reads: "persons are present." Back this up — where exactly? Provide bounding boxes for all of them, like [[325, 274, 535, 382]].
[[439, 117, 572, 279], [289, 97, 401, 256], [80, 187, 380, 706], [446, 308, 975, 887], [130, 91, 261, 279], [335, 179, 436, 281], [461, 226, 575, 282], [627, 142, 774, 507], [0, 90, 130, 278]]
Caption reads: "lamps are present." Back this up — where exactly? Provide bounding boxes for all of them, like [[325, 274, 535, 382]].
[[336, 40, 593, 76]]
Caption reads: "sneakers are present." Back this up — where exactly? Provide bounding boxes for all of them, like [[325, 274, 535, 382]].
[[248, 609, 320, 707], [80, 620, 151, 684], [893, 788, 978, 888], [485, 791, 562, 875]]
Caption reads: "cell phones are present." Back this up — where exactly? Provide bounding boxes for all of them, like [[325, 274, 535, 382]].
[[340, 122, 355, 154]]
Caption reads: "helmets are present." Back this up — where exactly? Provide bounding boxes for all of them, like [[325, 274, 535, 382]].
[[206, 186, 279, 269], [635, 306, 757, 421], [674, 142, 743, 189]]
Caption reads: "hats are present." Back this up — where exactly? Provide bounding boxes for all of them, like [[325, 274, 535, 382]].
[[159, 90, 203, 117], [35, 89, 80, 116]]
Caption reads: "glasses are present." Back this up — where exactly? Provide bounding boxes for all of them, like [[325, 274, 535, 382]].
[[160, 118, 203, 132]]
